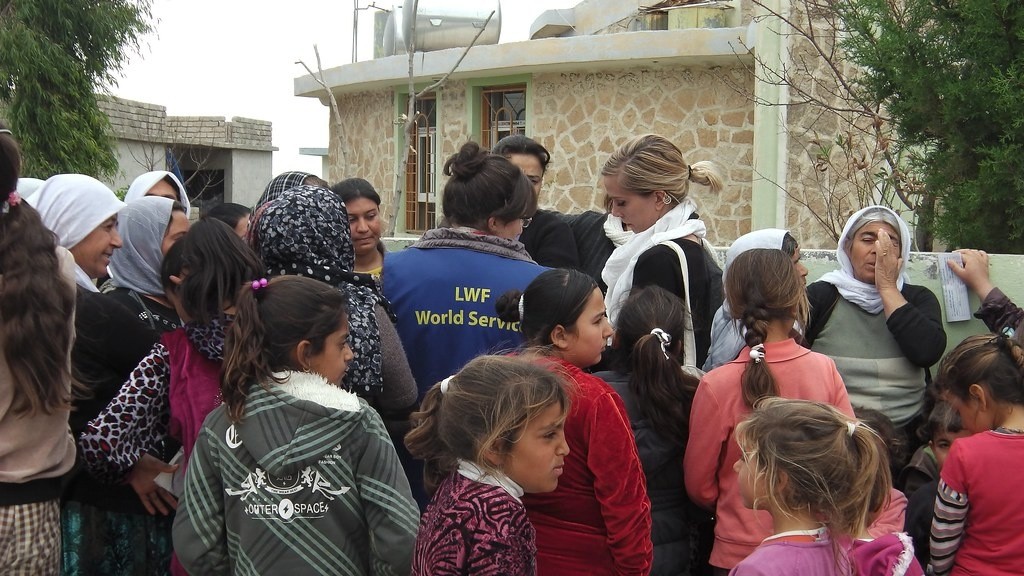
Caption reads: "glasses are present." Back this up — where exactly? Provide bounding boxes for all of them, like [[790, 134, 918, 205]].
[[518, 216, 532, 228]]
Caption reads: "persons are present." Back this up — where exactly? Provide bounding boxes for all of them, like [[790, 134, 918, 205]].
[[0, 129, 1024, 576]]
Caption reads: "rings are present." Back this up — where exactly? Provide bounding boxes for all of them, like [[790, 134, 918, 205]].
[[883, 253, 887, 256]]
